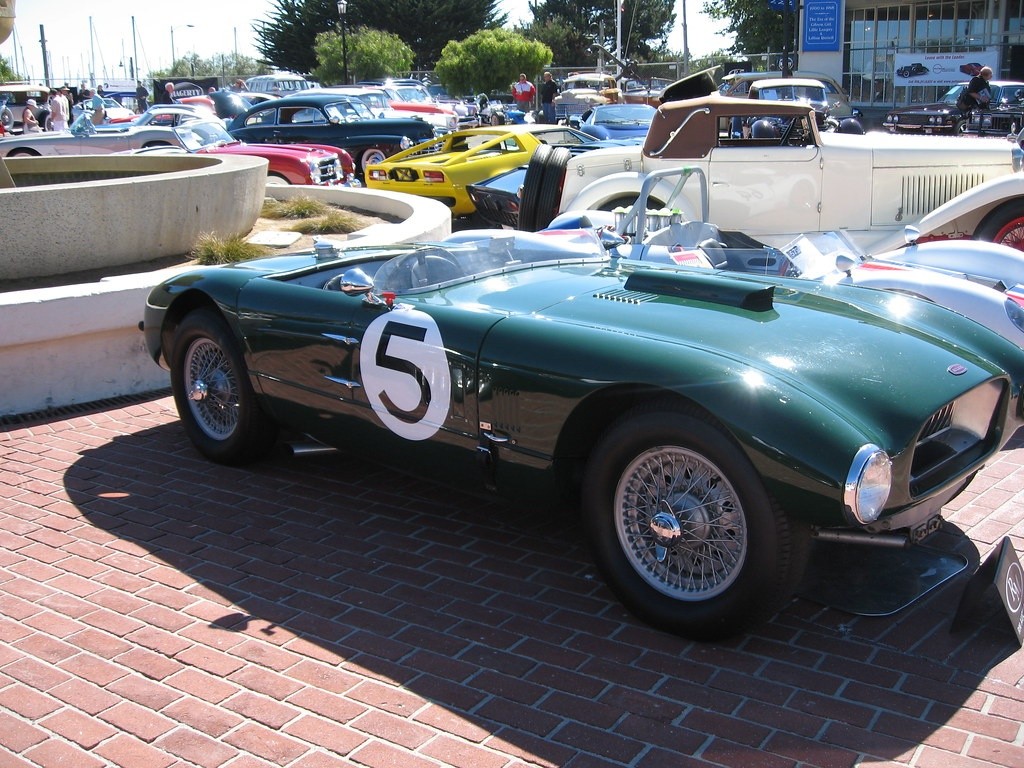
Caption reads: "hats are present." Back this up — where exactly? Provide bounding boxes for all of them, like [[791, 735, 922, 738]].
[[27, 99, 38, 108], [62, 87, 70, 91]]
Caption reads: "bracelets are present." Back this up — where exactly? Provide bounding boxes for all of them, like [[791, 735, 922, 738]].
[[979, 96, 983, 100]]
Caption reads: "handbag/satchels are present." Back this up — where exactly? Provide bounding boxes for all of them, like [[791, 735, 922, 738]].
[[956, 77, 979, 112]]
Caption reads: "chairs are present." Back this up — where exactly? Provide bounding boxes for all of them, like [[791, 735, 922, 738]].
[[322, 247, 482, 291], [482, 141, 500, 150]]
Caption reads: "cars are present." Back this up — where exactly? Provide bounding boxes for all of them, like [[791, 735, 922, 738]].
[[0, 74, 1024, 351]]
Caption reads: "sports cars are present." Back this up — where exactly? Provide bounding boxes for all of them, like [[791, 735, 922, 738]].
[[959, 63, 985, 76], [896, 63, 929, 78], [138, 165, 1024, 643]]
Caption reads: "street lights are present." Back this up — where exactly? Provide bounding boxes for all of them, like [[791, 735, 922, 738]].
[[337, 0, 348, 85], [170, 24, 194, 69]]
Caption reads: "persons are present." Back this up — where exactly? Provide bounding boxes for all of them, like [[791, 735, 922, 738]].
[[39, 81, 106, 131], [540, 72, 558, 124], [160, 83, 183, 104], [135, 80, 150, 114], [965, 66, 992, 124], [835, 118, 865, 135], [473, 93, 489, 113], [209, 87, 217, 93], [23, 100, 43, 134], [230, 80, 250, 93], [749, 120, 776, 138], [0, 95, 11, 137], [512, 74, 536, 113]]
